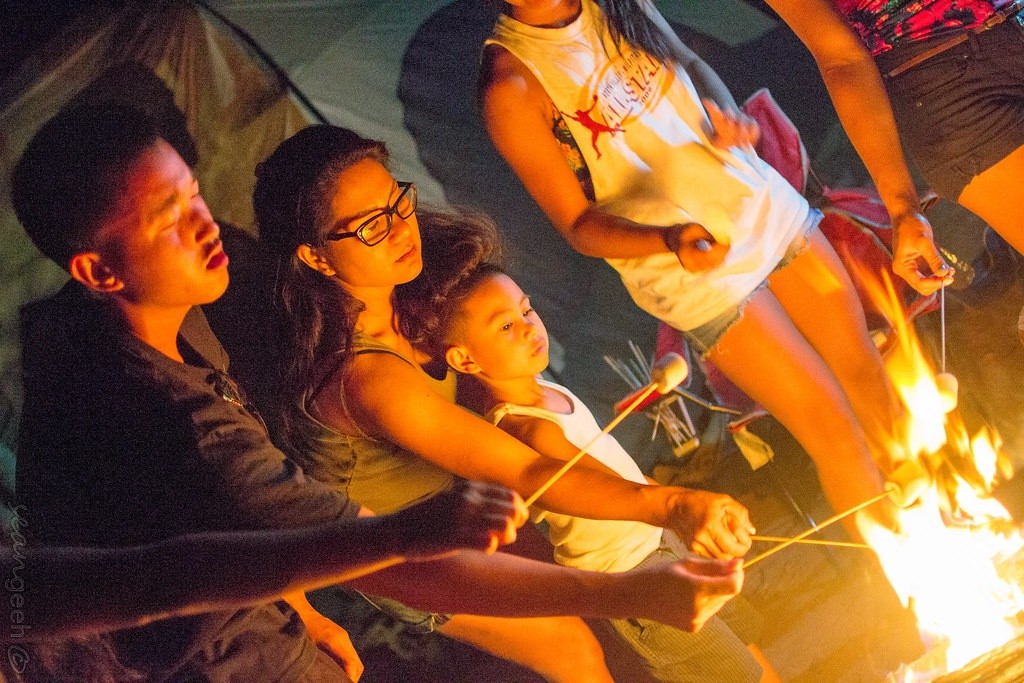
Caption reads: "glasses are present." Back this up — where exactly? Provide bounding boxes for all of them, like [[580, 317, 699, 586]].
[[320, 181, 418, 247]]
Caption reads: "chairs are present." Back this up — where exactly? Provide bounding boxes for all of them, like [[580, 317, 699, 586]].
[[651, 87, 944, 535]]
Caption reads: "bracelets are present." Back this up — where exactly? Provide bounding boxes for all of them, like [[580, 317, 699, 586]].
[[665, 223, 682, 251]]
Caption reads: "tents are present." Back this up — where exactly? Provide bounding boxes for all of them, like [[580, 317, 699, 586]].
[[0, 0, 1011, 659]]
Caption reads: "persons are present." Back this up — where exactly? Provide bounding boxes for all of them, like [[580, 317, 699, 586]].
[[764, 0, 1024, 296], [0, 102, 782, 683], [479, 0, 914, 568]]
